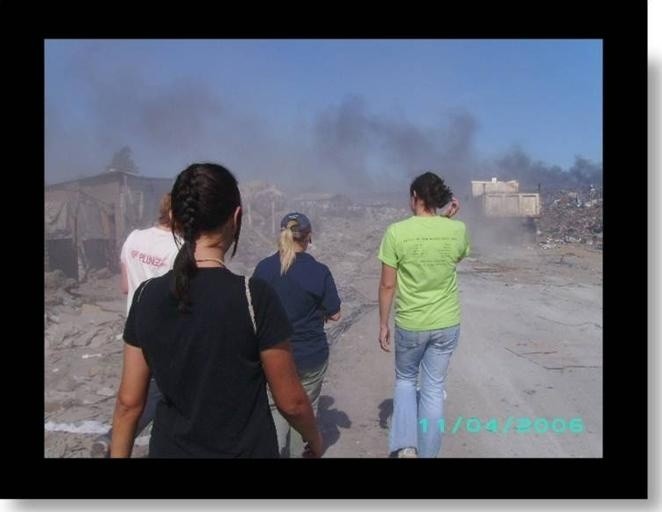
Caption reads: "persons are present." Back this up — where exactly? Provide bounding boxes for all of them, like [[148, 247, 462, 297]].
[[374, 170, 471, 457], [249, 211, 345, 458], [90, 190, 182, 460], [108, 161, 325, 458]]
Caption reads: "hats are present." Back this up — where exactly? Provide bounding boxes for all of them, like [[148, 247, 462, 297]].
[[280, 212, 312, 244]]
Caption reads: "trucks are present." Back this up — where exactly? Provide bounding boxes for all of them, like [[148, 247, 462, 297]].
[[465, 177, 541, 244]]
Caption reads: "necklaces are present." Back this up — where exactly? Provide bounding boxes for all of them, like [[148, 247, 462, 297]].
[[193, 257, 228, 266]]
